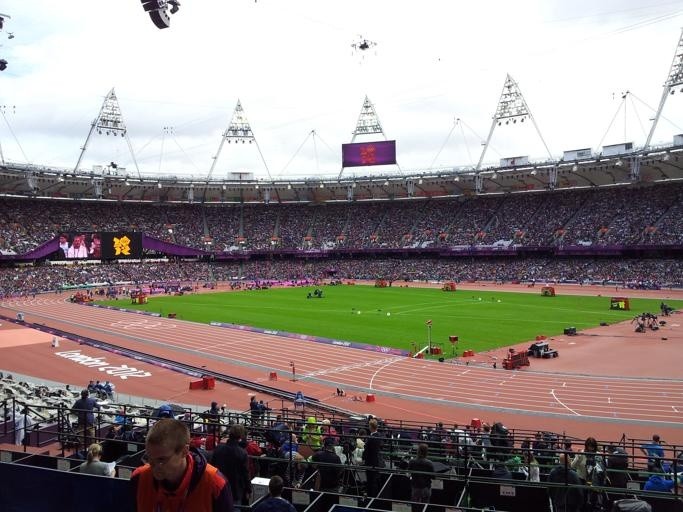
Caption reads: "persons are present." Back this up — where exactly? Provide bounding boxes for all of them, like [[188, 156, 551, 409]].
[[1, 374, 682, 512], [631, 302, 673, 332], [1, 183, 683, 305]]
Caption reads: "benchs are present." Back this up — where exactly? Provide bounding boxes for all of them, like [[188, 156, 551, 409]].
[[0, 378, 683, 511]]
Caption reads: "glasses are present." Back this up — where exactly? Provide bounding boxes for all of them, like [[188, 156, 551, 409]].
[[141, 452, 175, 465]]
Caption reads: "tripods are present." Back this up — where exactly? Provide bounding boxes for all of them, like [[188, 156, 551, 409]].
[[646, 317, 658, 326]]
